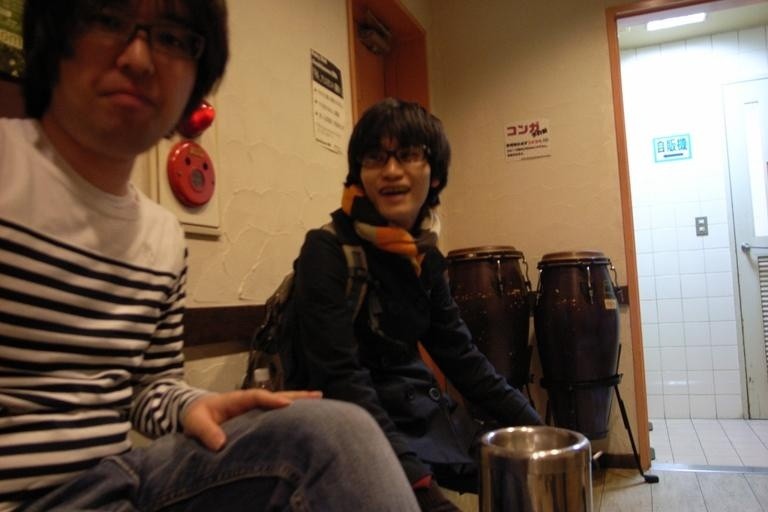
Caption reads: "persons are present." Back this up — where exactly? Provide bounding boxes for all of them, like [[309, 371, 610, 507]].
[[278, 97, 548, 512], [1, 0, 422, 512]]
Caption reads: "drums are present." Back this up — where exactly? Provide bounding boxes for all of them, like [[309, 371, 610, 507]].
[[442, 247, 536, 410], [534, 250, 623, 477]]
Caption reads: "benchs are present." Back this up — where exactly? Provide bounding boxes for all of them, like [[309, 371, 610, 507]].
[[181, 304, 268, 349]]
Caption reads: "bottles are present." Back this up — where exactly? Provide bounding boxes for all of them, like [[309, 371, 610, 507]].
[[250, 367, 274, 393]]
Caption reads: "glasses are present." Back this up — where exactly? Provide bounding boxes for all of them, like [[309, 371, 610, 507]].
[[353, 139, 431, 168], [72, 12, 207, 63]]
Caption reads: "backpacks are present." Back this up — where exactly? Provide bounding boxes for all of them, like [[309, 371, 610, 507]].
[[242, 270, 297, 393]]
